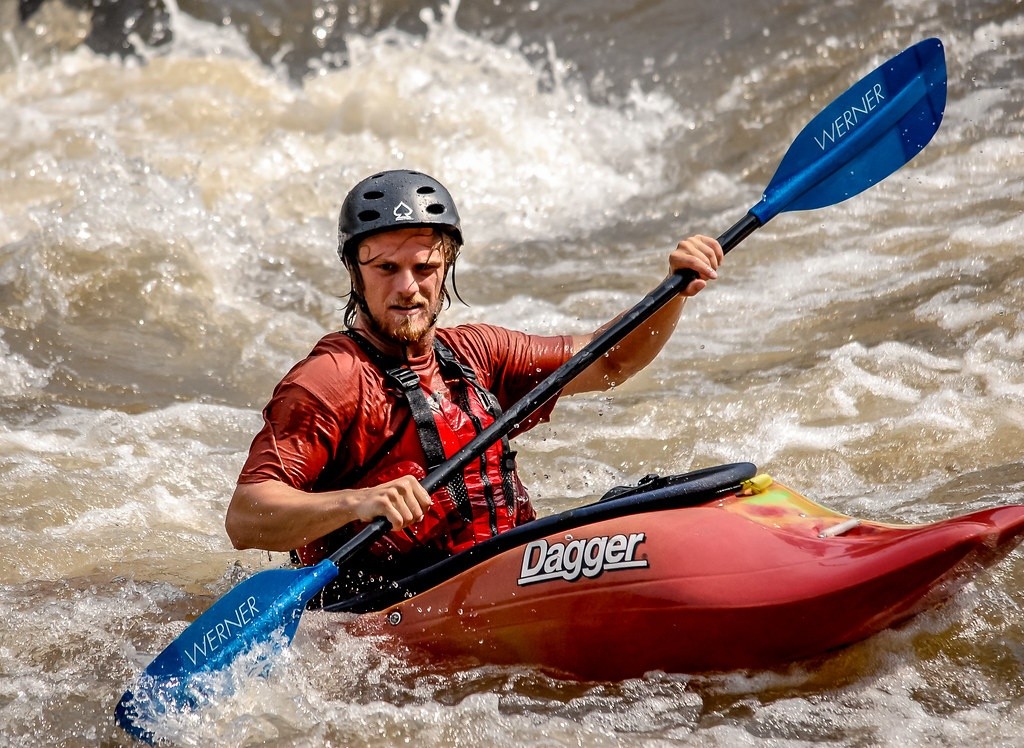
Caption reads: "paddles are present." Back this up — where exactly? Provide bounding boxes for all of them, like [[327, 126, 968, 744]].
[[112, 37, 950, 745]]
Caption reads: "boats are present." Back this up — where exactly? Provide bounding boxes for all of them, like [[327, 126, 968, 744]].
[[311, 462, 1024, 680]]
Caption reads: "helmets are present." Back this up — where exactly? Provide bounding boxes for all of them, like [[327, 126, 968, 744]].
[[336, 169, 463, 260]]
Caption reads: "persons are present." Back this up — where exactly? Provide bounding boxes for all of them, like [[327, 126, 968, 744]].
[[224, 169, 724, 612]]
